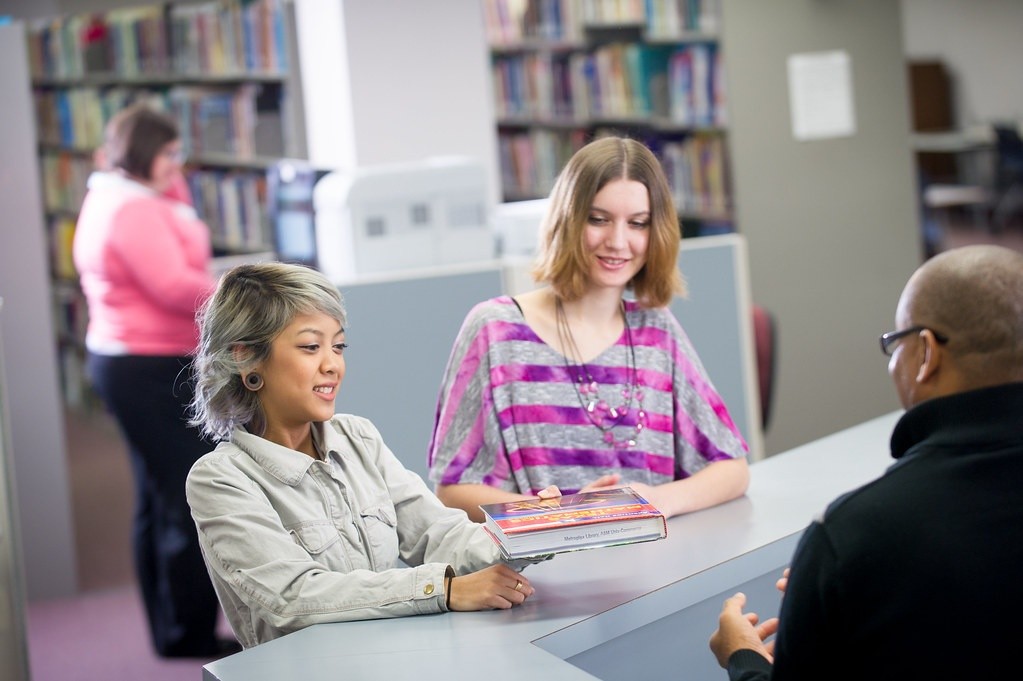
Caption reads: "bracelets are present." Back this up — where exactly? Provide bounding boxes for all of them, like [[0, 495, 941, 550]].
[[444, 576, 452, 608]]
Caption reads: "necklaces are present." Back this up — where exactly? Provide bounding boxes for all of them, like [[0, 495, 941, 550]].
[[555, 296, 646, 449]]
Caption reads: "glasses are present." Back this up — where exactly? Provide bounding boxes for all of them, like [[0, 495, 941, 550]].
[[881, 324, 950, 356]]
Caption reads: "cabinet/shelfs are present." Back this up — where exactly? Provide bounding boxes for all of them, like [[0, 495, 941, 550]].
[[0, 1, 924, 610]]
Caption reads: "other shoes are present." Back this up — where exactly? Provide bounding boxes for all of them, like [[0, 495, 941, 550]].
[[161, 635, 244, 661]]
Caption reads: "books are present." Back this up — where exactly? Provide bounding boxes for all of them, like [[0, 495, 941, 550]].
[[479, 486, 667, 561], [17, 2, 286, 404], [479, 2, 735, 240]]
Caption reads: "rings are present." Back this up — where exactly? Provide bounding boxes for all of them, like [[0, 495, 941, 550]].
[[515, 579, 522, 590]]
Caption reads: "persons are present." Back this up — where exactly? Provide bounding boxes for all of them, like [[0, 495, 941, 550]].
[[186, 259, 535, 650], [71, 103, 241, 660], [711, 241, 1022, 681], [423, 135, 749, 520]]
